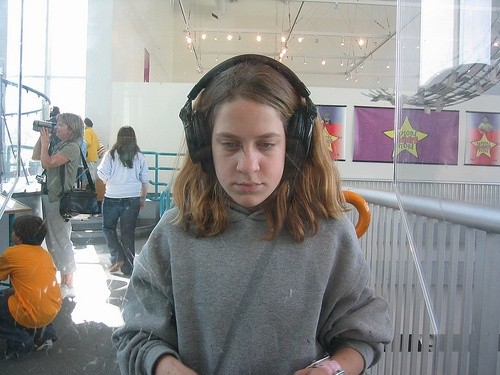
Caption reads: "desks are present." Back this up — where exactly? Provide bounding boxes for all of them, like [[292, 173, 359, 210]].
[[0, 194, 32, 294]]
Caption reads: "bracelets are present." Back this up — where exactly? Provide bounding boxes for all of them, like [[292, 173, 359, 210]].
[[307, 355, 344, 375]]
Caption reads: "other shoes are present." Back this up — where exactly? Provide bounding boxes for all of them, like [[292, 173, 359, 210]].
[[60, 282, 75, 300], [107, 259, 124, 272], [34, 338, 53, 352]]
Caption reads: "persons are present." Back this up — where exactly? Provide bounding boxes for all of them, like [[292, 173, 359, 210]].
[[0, 215, 63, 359], [97, 126, 149, 276], [32, 113, 84, 300], [111, 53, 393, 375], [79, 118, 107, 191]]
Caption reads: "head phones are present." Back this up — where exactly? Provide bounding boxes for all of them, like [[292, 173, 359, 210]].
[[179, 54, 318, 167]]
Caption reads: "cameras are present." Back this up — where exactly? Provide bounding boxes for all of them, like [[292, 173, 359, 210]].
[[36, 174, 46, 184]]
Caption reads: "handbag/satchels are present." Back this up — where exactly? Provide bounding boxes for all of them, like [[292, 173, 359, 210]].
[[60, 141, 101, 219], [97, 140, 107, 158]]
